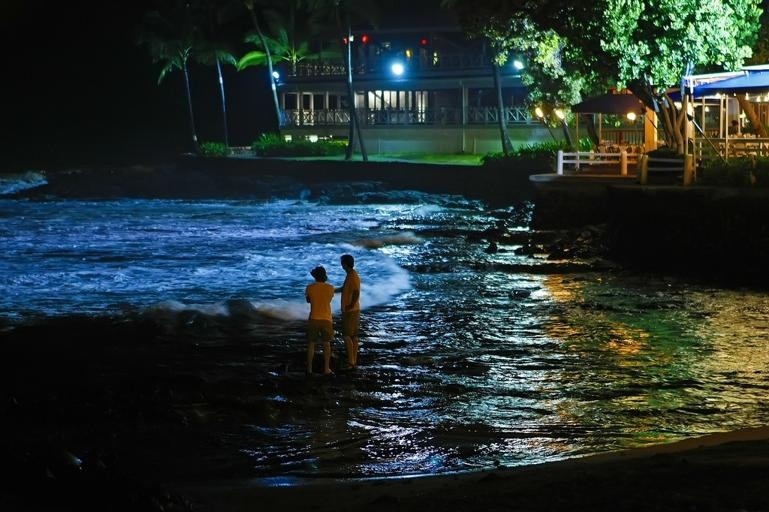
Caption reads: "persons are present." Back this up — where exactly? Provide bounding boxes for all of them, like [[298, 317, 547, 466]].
[[334, 253, 362, 372], [724, 120, 739, 135], [301, 265, 335, 374]]
